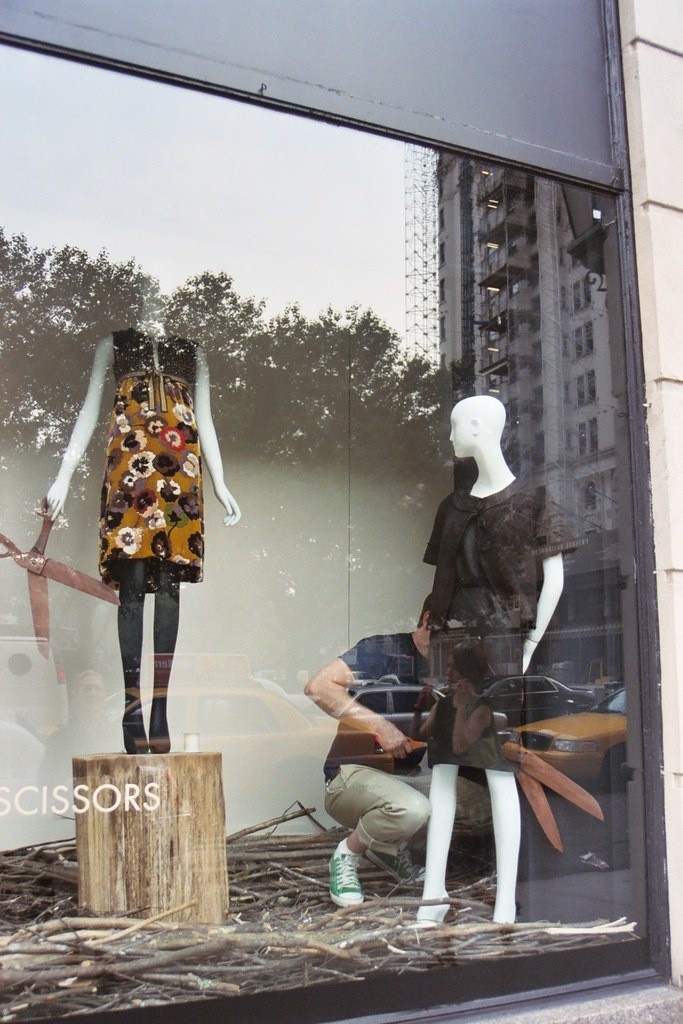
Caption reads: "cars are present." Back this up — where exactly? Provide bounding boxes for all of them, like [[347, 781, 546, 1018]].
[[472, 676, 598, 730], [313, 684, 506, 738], [503, 683, 629, 790], [54, 683, 397, 802]]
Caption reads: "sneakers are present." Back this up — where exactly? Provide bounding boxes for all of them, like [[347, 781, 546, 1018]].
[[327, 849, 362, 907], [367, 843, 426, 885]]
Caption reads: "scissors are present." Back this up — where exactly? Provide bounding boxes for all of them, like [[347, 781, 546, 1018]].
[[0, 496, 120, 659], [406, 737, 427, 751], [514, 737, 604, 853]]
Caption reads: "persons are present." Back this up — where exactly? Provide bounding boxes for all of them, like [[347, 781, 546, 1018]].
[[409, 640, 498, 762], [303, 594, 442, 908], [45, 275, 240, 754], [414, 395, 582, 930]]
